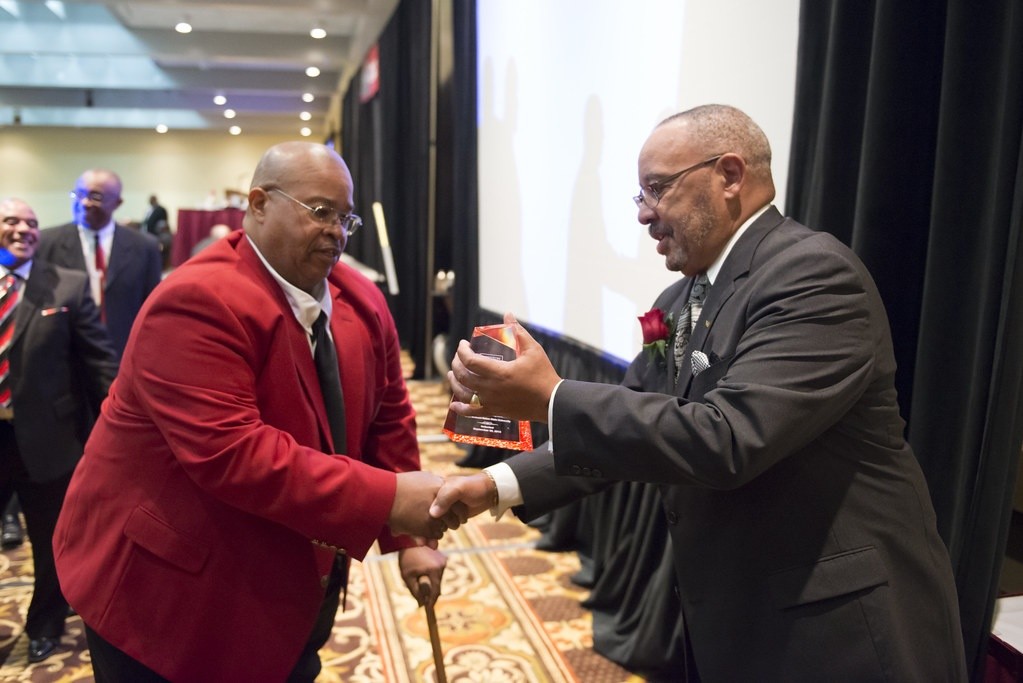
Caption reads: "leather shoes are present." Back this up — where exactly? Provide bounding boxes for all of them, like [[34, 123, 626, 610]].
[[65, 607, 78, 616], [28, 635, 56, 661], [1, 514, 21, 544]]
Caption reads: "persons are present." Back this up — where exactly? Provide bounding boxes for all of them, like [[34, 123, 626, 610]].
[[0, 197, 118, 662], [52, 140, 469, 683], [144, 196, 172, 272], [33, 168, 161, 363], [392, 104, 968, 683]]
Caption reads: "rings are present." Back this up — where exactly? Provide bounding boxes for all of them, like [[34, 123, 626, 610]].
[[469, 391, 481, 407]]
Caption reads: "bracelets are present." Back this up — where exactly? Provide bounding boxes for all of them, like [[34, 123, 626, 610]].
[[482, 469, 497, 514]]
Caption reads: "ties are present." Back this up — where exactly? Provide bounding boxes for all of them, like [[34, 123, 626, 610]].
[[311, 311, 343, 456], [93, 235, 107, 325], [674, 282, 707, 375], [0, 273, 18, 408]]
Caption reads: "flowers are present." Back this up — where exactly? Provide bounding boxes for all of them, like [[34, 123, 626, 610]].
[[638, 308, 677, 370]]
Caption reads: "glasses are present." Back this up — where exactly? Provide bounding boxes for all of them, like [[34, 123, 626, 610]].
[[70, 190, 115, 204], [631, 153, 720, 209], [265, 187, 361, 235]]
